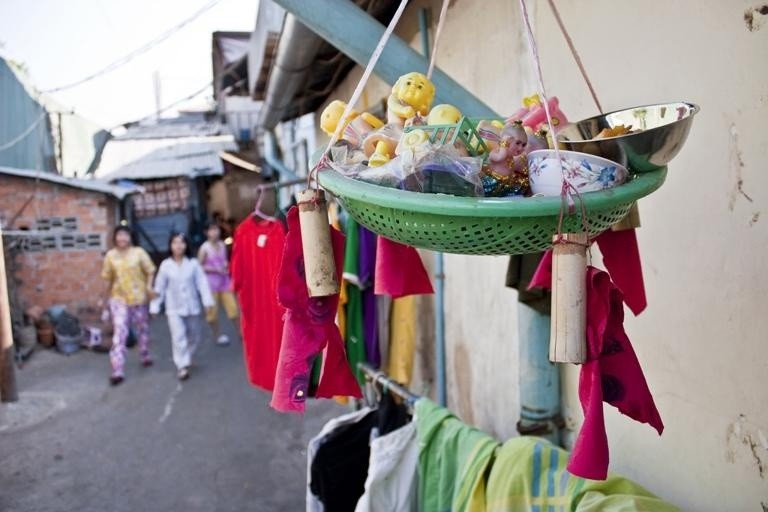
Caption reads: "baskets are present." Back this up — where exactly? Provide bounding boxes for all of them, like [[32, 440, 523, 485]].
[[307, 145, 669, 258]]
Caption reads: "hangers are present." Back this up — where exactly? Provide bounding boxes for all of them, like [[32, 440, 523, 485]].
[[316, 371, 424, 445], [234, 181, 297, 233]]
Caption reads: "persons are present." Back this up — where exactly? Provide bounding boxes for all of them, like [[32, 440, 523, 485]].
[[146, 230, 219, 382], [197, 221, 244, 346], [211, 212, 232, 239], [507, 135, 550, 195], [486, 124, 525, 196], [98, 222, 159, 384]]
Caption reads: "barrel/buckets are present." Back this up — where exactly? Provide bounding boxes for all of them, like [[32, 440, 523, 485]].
[[54, 328, 88, 352]]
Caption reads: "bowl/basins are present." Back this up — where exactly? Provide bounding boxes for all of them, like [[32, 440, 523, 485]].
[[554, 102, 701, 172], [524, 148, 629, 197]]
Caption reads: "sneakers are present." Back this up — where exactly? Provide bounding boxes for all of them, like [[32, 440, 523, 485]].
[[177, 368, 189, 380], [216, 333, 231, 344], [109, 377, 123, 385]]
